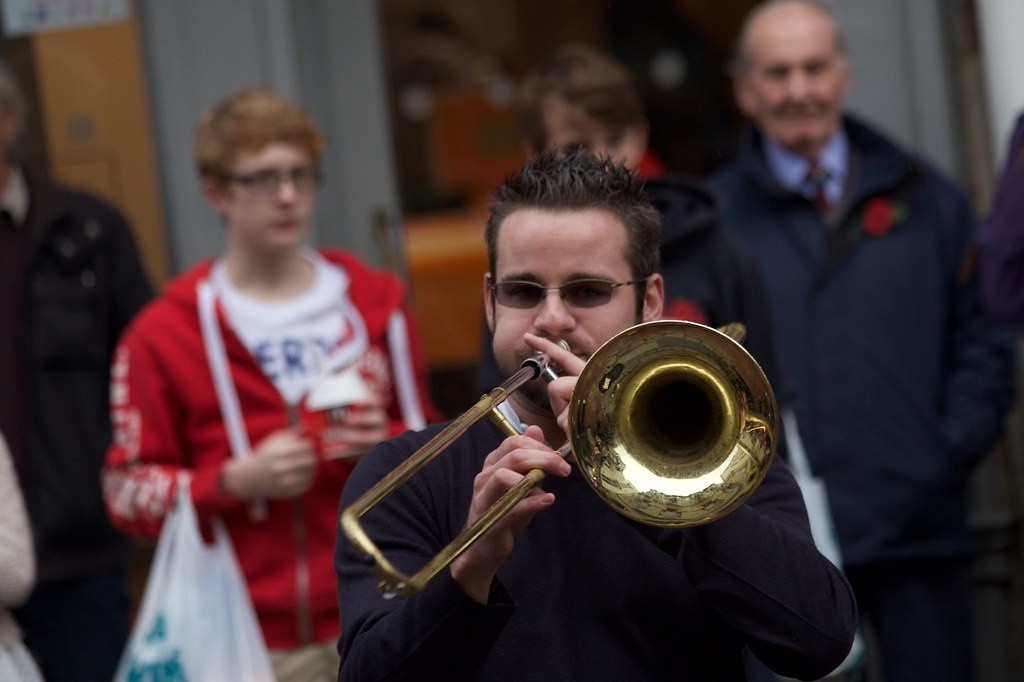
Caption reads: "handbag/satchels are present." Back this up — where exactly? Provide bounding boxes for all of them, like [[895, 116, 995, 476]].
[[114, 477, 279, 682]]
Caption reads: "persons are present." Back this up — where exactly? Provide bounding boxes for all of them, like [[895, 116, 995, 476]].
[[520, 46, 706, 325], [0, 66, 162, 681], [683, 0, 1012, 682], [336, 146, 856, 682], [0, 424, 51, 680], [102, 88, 450, 682], [970, 97, 1024, 392]]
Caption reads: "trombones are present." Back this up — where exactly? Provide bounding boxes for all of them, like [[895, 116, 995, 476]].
[[341, 322, 780, 600]]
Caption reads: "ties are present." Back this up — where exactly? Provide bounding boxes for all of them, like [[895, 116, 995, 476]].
[[804, 163, 833, 216]]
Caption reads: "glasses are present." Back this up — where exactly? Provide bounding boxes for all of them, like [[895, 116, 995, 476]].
[[225, 171, 328, 193], [486, 273, 647, 310]]
